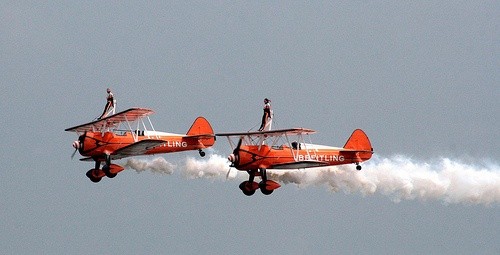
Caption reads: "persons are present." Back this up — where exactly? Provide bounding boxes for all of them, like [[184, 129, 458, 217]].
[[257, 97, 274, 131], [97, 88, 116, 120]]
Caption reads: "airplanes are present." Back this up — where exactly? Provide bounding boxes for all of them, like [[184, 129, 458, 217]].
[[66, 107, 217, 185], [182, 127, 374, 195]]
